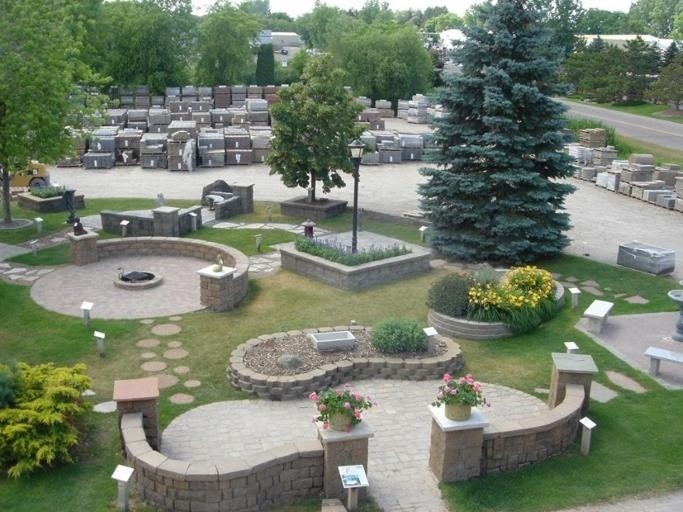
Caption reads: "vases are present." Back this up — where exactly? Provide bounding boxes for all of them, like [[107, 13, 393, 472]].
[[328, 408, 354, 433], [445, 400, 472, 422]]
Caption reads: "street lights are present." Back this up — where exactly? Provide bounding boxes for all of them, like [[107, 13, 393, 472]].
[[348, 139, 366, 253]]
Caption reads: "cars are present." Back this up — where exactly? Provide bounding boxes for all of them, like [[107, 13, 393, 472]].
[[9, 160, 50, 187]]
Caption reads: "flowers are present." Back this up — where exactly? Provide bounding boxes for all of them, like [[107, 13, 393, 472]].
[[307, 385, 373, 433], [424, 372, 488, 407]]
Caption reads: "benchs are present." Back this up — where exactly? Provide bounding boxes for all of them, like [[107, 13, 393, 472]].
[[641, 345, 683, 377]]
